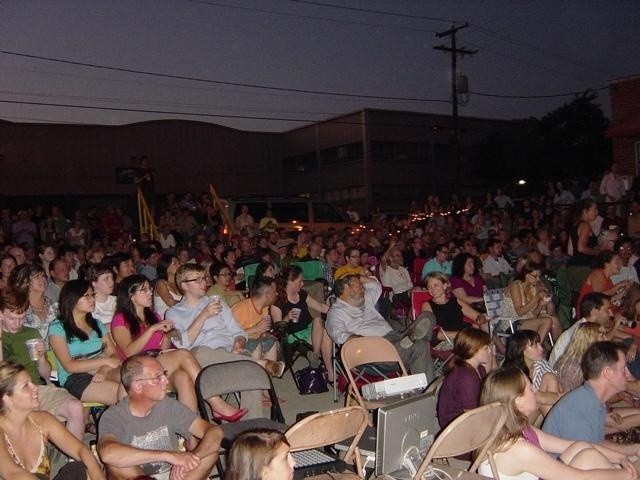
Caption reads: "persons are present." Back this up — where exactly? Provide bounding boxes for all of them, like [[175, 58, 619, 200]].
[[1, 359, 107, 479], [436, 179, 573, 252], [290, 214, 435, 245], [477, 368, 639, 479], [543, 341, 639, 462], [86, 264, 118, 335], [279, 265, 337, 385], [227, 430, 295, 479], [111, 274, 249, 452], [327, 273, 435, 390], [500, 263, 560, 346], [423, 272, 488, 361], [576, 251, 619, 315], [451, 252, 486, 313], [437, 329, 491, 461], [99, 356, 223, 479], [14, 265, 62, 339], [336, 247, 369, 280], [603, 204, 625, 237], [614, 280, 639, 339], [422, 246, 451, 279], [1, 288, 52, 387], [549, 292, 614, 367], [611, 234, 639, 283], [500, 331, 564, 427], [164, 263, 286, 378], [45, 278, 125, 404], [602, 163, 624, 225], [381, 244, 412, 318], [206, 263, 244, 306], [553, 322, 639, 406], [568, 196, 602, 303], [233, 278, 279, 361], [1, 191, 288, 276]]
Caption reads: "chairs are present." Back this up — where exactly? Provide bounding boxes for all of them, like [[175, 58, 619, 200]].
[[484, 286, 555, 364], [411, 289, 483, 374], [194, 359, 293, 480], [340, 335, 422, 411], [413, 401, 510, 480], [411, 258, 428, 286], [283, 405, 370, 480]]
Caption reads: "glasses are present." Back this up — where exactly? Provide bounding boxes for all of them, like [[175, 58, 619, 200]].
[[620, 247, 635, 252], [182, 275, 209, 283], [83, 292, 97, 299], [529, 273, 543, 280], [218, 272, 234, 277], [135, 370, 168, 382], [349, 255, 362, 259], [30, 275, 48, 280], [137, 287, 153, 294]]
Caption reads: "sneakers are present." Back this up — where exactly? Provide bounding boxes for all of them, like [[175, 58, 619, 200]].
[[264, 359, 285, 377]]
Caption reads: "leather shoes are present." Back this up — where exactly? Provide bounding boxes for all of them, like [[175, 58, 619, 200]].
[[399, 311, 435, 349]]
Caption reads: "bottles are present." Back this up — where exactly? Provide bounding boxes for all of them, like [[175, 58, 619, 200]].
[[536, 281, 552, 303]]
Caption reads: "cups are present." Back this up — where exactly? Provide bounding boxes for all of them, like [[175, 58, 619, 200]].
[[25, 338, 43, 361], [263, 315, 270, 321], [291, 308, 301, 323], [209, 295, 221, 314]]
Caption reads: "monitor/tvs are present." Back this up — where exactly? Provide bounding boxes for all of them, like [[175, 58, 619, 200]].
[[375, 392, 444, 480]]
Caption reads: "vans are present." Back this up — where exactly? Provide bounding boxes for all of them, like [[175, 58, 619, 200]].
[[229, 199, 355, 232]]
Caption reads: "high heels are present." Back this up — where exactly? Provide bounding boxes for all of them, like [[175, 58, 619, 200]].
[[213, 408, 248, 424]]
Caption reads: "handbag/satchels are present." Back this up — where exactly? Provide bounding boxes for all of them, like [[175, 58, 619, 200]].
[[406, 447, 434, 480], [298, 367, 329, 395]]
